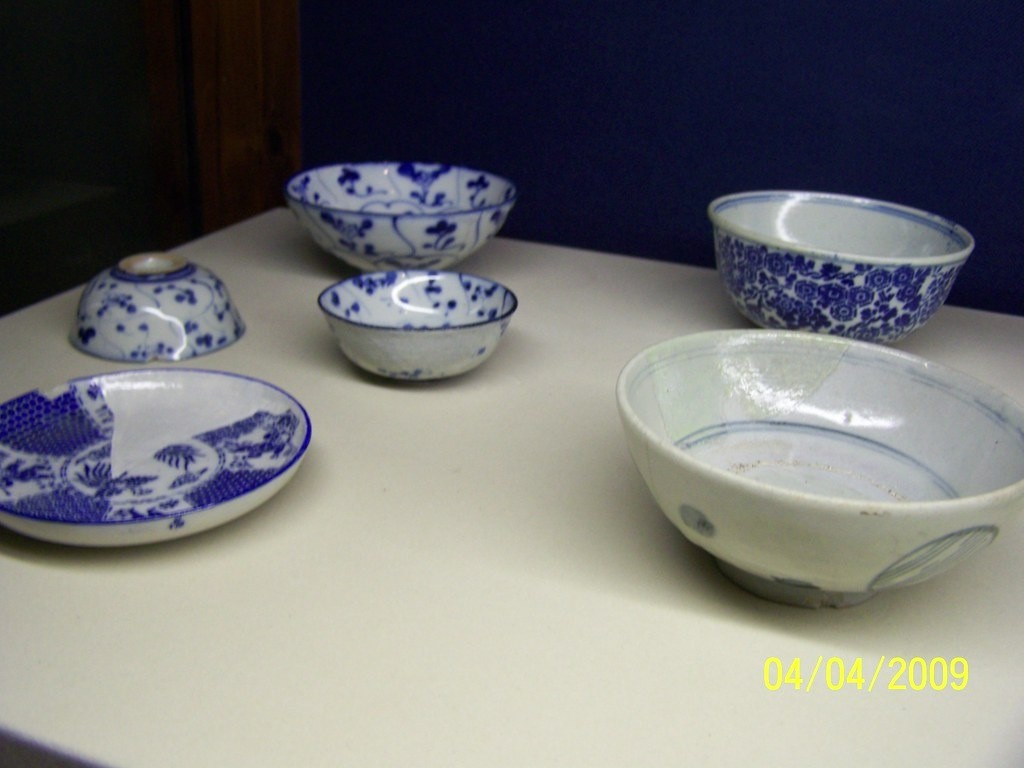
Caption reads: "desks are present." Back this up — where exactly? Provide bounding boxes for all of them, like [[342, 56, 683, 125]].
[[0, 205, 1024, 768]]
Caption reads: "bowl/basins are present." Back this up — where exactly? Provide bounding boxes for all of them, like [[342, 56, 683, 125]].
[[317, 267, 518, 381], [67, 251, 247, 364], [615, 329, 1024, 612], [708, 189, 975, 345], [286, 161, 518, 274]]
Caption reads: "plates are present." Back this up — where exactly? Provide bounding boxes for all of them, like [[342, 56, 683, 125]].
[[0, 368, 311, 548]]
